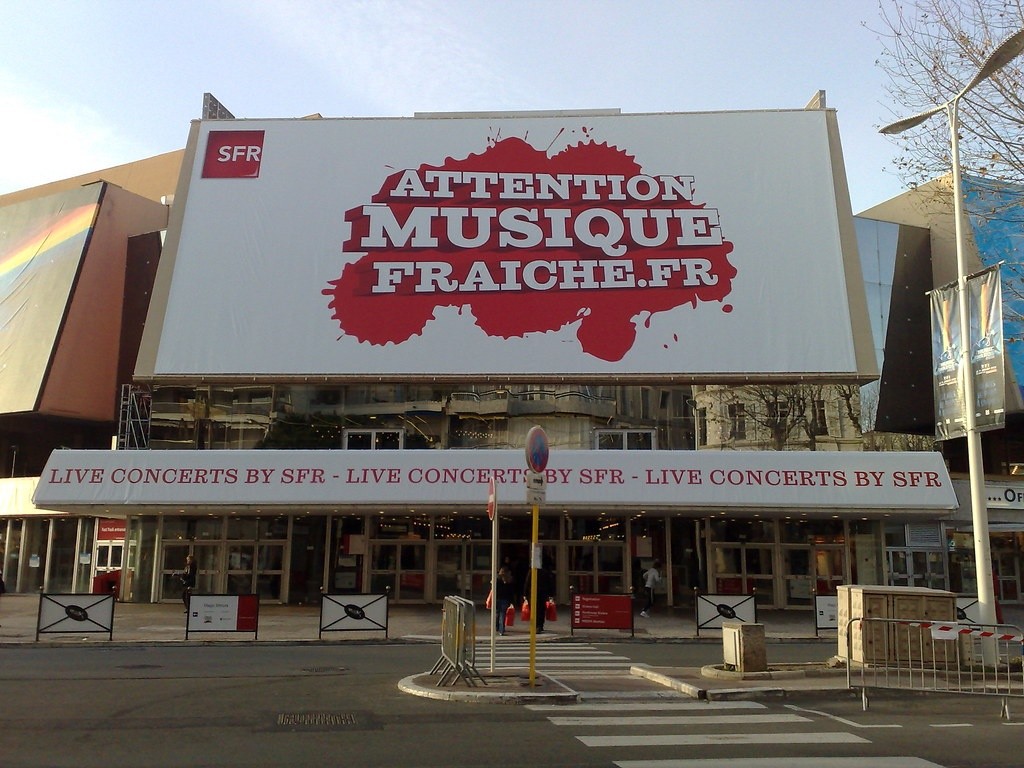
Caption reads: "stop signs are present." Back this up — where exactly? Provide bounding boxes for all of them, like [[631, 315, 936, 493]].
[[488, 475, 495, 520]]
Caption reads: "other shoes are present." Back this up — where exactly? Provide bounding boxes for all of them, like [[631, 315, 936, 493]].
[[536, 627, 545, 634], [639, 612, 649, 618], [498, 631, 503, 636]]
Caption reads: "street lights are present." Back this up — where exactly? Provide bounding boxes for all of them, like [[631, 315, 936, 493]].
[[879, 23, 1024, 671]]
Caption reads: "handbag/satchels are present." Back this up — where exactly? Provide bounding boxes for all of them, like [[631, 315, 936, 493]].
[[505, 604, 516, 626], [546, 599, 557, 621], [485, 589, 493, 609], [520, 599, 529, 621]]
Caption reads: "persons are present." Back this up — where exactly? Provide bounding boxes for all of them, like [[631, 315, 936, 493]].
[[523, 568, 550, 634], [495, 568, 512, 636], [640, 563, 663, 617], [183, 555, 197, 613]]
